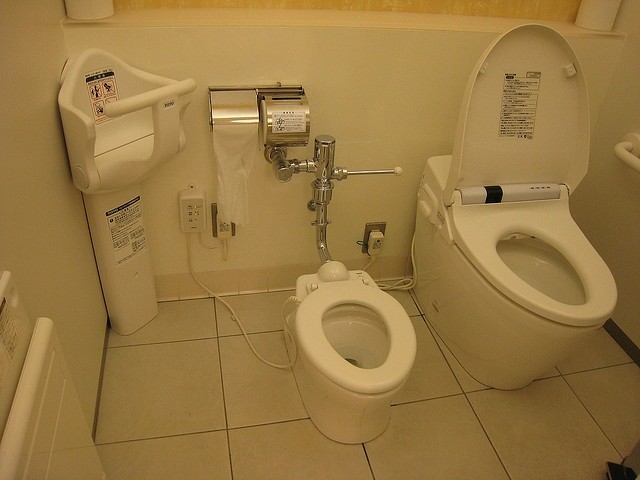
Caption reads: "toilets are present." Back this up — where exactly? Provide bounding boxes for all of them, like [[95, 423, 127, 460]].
[[283, 134, 417, 446], [407, 20, 620, 392]]
